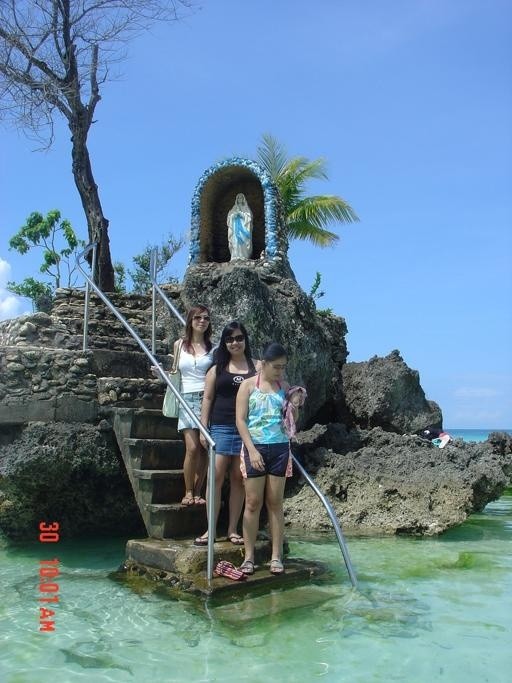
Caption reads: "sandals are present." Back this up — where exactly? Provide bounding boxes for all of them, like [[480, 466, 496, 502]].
[[239, 558, 256, 574], [269, 559, 285, 574]]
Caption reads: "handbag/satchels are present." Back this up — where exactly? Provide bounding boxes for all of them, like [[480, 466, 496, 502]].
[[161, 338, 183, 419]]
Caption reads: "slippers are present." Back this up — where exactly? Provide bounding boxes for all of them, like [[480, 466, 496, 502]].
[[214, 560, 243, 580], [180, 495, 195, 506], [192, 533, 217, 546], [227, 534, 247, 545], [195, 495, 207, 506]]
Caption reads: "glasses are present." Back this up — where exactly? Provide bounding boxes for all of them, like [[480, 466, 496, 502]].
[[192, 315, 211, 322], [268, 361, 288, 370], [224, 335, 245, 343]]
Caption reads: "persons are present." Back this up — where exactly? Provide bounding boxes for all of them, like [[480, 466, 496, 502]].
[[234, 343, 309, 575], [150, 304, 215, 506], [226, 192, 253, 262], [192, 322, 261, 546]]
